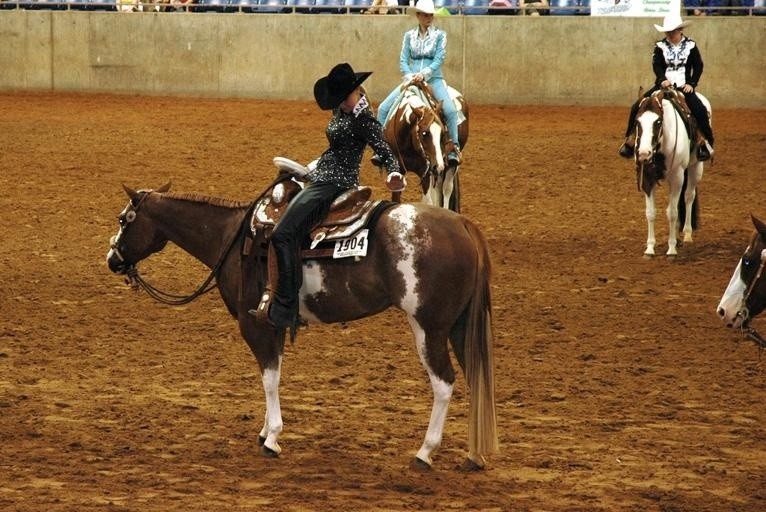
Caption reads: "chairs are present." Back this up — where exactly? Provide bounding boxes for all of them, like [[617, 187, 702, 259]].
[[0, 0, 589, 16]]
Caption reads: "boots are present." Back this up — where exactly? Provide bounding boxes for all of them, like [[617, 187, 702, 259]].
[[696, 131, 713, 161], [446, 143, 462, 164], [371, 153, 384, 167], [620, 134, 637, 158], [248, 288, 299, 330]]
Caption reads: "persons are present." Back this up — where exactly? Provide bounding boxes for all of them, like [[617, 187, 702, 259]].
[[247, 62, 407, 330], [619, 13, 714, 161], [370, 1, 461, 166]]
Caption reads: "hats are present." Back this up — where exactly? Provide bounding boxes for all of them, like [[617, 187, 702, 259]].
[[312, 62, 373, 110], [406, 0, 440, 17], [653, 12, 692, 32]]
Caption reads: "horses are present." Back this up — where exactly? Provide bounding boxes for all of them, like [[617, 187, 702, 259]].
[[104, 179, 497, 472], [381, 79, 471, 215], [629, 84, 714, 261], [714, 210, 766, 337]]
